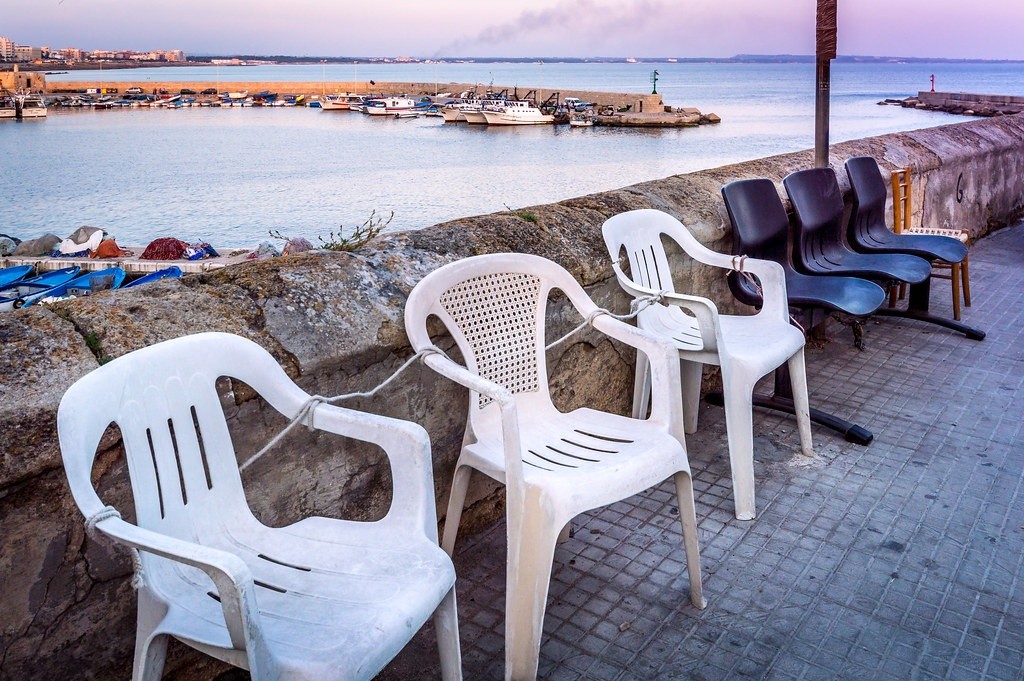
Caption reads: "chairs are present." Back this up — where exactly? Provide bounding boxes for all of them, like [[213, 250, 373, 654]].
[[404, 252, 708, 681], [56, 331, 466, 681], [844, 156, 986, 341], [704, 176, 885, 446], [782, 167, 931, 285], [600, 207, 815, 520], [888, 165, 972, 322]]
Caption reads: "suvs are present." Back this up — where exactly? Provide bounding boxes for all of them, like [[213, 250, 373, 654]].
[[564, 97, 592, 112]]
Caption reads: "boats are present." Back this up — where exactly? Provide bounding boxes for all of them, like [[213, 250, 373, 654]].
[[0, 265, 184, 313], [51, 95, 321, 109], [436, 79, 560, 125], [0, 87, 48, 117], [321, 92, 443, 118]]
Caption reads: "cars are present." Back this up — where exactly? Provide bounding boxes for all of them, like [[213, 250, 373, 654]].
[[125, 87, 144, 94]]
